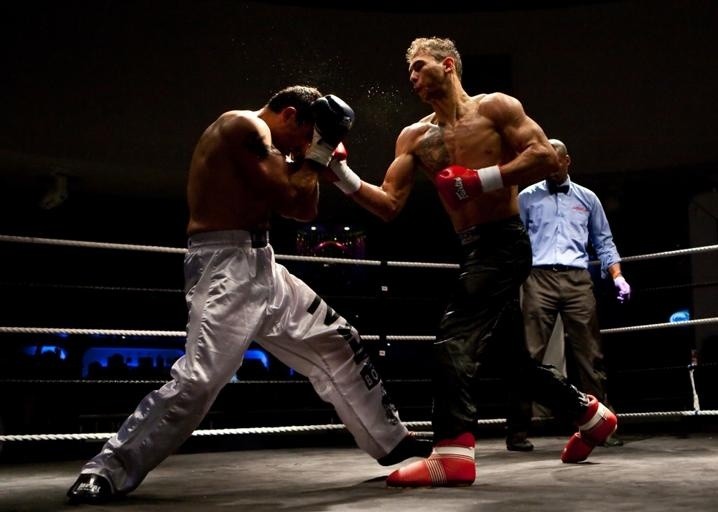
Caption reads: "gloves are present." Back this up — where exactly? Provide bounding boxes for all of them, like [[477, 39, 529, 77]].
[[304, 93, 356, 169], [614, 276, 631, 304], [432, 164, 505, 211], [290, 134, 361, 195]]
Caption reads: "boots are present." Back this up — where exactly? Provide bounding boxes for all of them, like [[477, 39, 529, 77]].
[[561, 394, 618, 462], [386, 432, 475, 486]]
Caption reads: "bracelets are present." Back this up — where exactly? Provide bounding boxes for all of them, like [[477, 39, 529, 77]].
[[612, 271, 622, 278]]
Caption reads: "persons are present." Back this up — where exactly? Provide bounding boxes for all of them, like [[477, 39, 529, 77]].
[[504, 137, 632, 451], [289, 35, 620, 492], [63, 86, 434, 506]]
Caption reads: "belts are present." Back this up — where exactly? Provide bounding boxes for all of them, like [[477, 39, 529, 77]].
[[541, 265, 576, 272]]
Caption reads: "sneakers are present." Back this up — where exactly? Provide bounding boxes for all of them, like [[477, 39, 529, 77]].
[[67, 473, 111, 504], [378, 434, 432, 466], [507, 439, 533, 451], [605, 435, 623, 446]]
